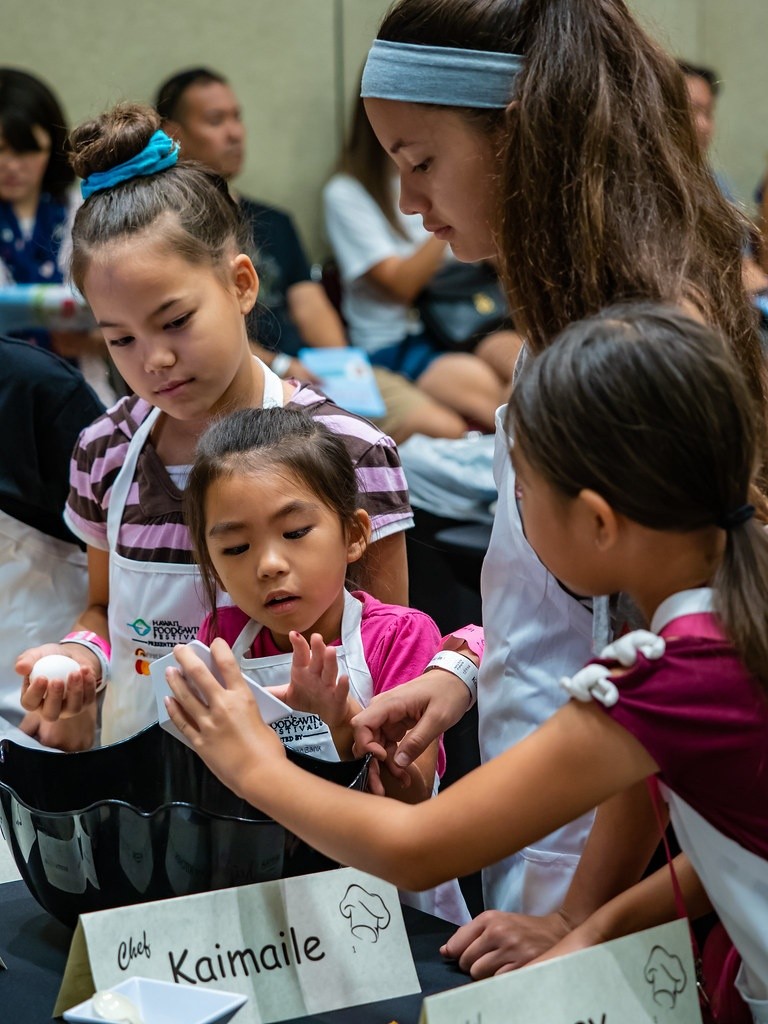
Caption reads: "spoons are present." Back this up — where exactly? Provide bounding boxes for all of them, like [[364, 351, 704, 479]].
[[93, 990, 144, 1024]]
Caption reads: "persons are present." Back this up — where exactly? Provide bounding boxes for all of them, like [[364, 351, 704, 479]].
[[156, 69, 466, 447], [1, 58, 767, 923], [350, 1, 767, 980], [161, 299, 767, 1024]]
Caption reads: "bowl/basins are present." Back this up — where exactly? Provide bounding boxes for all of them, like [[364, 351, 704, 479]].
[[0, 719, 373, 932], [63, 977, 247, 1024]]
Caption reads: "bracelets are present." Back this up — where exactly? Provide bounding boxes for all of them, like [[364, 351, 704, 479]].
[[439, 624, 485, 665], [62, 631, 111, 684], [423, 651, 478, 712], [271, 354, 291, 375], [59, 640, 110, 693]]
